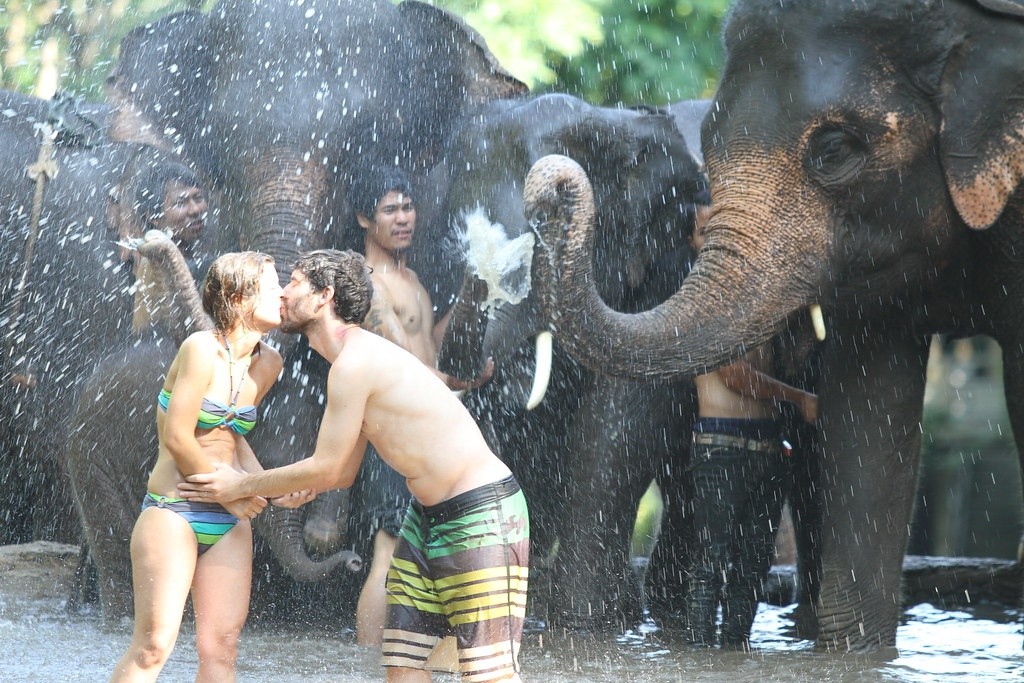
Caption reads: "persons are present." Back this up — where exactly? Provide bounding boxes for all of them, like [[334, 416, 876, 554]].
[[108, 168, 528, 683], [678, 190, 820, 652]]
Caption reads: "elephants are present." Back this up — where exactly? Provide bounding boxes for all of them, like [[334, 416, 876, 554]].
[[0, 0, 1024, 655]]
